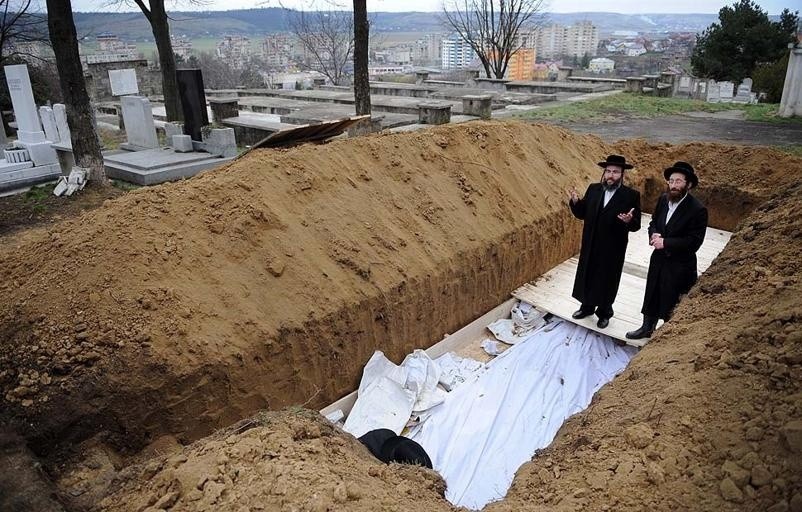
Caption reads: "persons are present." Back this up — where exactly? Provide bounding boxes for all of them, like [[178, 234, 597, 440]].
[[562, 155, 641, 330], [623, 160, 707, 340]]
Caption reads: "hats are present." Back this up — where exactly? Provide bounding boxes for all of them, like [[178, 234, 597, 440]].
[[596, 154, 634, 170]]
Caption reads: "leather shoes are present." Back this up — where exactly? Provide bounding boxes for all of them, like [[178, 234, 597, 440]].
[[625, 326, 651, 339], [596, 317, 609, 328], [572, 308, 595, 320]]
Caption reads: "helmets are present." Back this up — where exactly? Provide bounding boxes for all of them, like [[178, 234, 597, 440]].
[[663, 161, 699, 189]]
